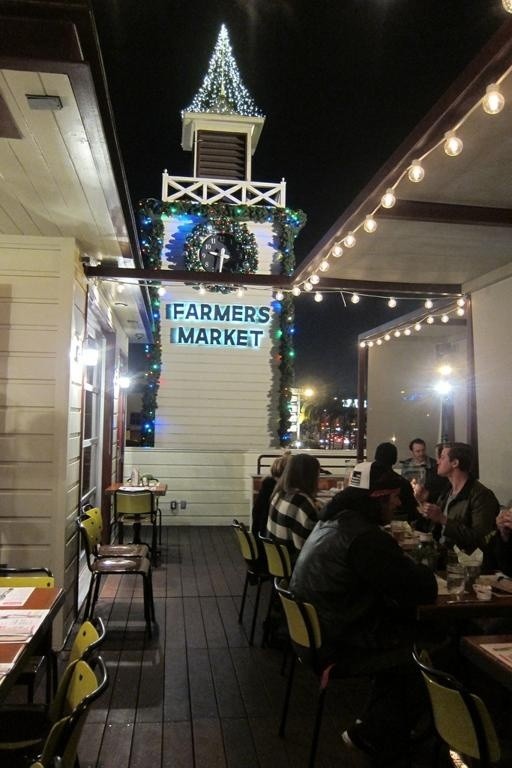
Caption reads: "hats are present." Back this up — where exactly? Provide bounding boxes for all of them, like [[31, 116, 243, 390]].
[[349, 462, 402, 489]]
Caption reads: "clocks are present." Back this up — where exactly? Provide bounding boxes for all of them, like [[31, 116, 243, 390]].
[[183, 218, 260, 296]]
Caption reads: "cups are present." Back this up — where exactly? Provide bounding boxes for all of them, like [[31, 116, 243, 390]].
[[444, 563, 466, 604], [389, 519, 433, 551], [336, 480, 344, 491], [463, 560, 482, 593], [141, 477, 149, 487], [472, 584, 493, 601]]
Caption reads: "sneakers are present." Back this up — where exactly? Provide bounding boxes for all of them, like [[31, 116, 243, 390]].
[[341, 717, 375, 758]]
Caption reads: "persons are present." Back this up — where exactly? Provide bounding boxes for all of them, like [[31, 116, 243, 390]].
[[262, 454, 326, 582], [410, 441, 501, 552], [408, 442, 449, 532], [345, 442, 422, 522], [402, 437, 437, 492], [251, 448, 292, 572], [480, 497, 512, 579], [284, 460, 439, 768]]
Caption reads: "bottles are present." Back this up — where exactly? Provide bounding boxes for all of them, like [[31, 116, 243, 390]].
[[130, 463, 140, 486], [343, 461, 354, 490]]
